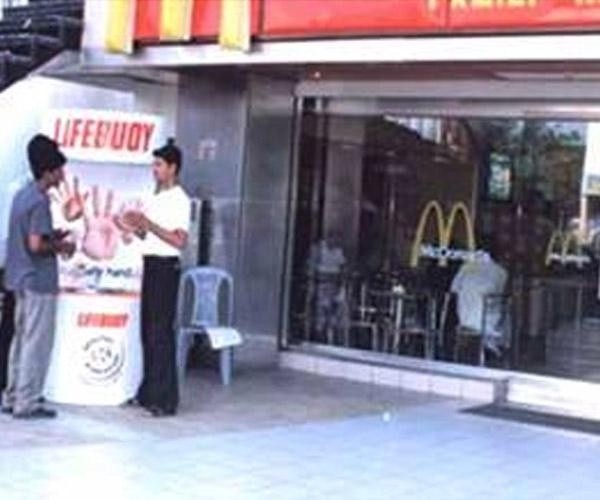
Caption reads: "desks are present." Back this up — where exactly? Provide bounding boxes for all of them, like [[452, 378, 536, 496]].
[[40, 283, 148, 408]]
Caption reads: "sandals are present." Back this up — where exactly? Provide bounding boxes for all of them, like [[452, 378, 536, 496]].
[[5, 401, 55, 419], [121, 395, 178, 416]]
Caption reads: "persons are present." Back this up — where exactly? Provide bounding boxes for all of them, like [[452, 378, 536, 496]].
[[112, 137, 192, 418], [0, 133, 77, 419]]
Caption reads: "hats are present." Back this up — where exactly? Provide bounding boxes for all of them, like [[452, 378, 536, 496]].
[[27, 134, 65, 173]]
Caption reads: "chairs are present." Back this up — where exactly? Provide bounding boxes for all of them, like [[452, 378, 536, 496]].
[[305, 264, 517, 373], [178, 263, 237, 387]]
